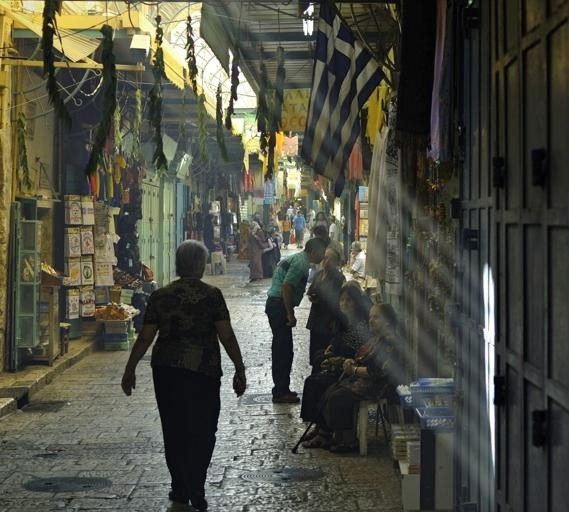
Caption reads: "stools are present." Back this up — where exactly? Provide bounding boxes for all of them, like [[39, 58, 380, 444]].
[[354, 398, 387, 456]]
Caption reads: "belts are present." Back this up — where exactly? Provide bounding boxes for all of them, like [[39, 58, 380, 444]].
[[358, 277, 364, 280]]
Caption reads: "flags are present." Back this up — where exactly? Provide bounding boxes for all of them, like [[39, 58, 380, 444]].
[[300, 0, 385, 198]]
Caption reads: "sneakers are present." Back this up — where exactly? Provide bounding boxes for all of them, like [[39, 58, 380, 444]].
[[301, 437, 329, 448], [188, 491, 209, 510], [290, 391, 297, 396], [329, 441, 360, 457], [272, 396, 300, 403], [168, 491, 187, 505], [302, 429, 317, 440]]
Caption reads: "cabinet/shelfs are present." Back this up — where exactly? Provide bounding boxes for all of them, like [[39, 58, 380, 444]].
[[24, 284, 61, 366]]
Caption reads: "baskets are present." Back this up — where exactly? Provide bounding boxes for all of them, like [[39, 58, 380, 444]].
[[397, 378, 456, 408], [416, 406, 457, 433]]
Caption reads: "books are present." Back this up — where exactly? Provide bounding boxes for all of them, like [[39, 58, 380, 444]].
[[64, 193, 113, 320]]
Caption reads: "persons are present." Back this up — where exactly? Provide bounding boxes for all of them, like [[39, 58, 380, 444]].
[[121, 240, 246, 510], [248, 203, 402, 453], [204, 214, 216, 264]]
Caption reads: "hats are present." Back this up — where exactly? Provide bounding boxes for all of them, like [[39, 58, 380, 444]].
[[349, 241, 361, 250]]
